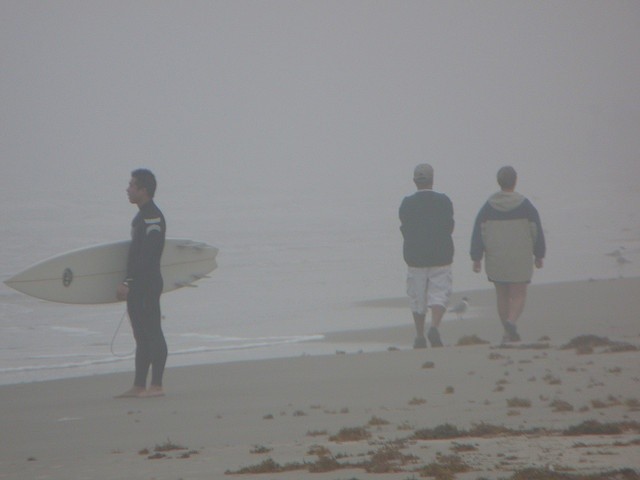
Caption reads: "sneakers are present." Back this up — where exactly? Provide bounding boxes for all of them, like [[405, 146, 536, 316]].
[[413, 337, 426, 348], [504, 320, 520, 342], [427, 327, 443, 347]]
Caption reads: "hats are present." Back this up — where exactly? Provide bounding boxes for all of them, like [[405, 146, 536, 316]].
[[413, 164, 433, 183]]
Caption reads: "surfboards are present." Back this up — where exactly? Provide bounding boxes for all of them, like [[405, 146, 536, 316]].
[[3, 238, 219, 304]]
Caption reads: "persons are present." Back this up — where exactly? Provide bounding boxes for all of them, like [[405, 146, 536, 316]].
[[399, 163, 456, 349], [469, 167, 547, 343], [115, 169, 168, 398]]
